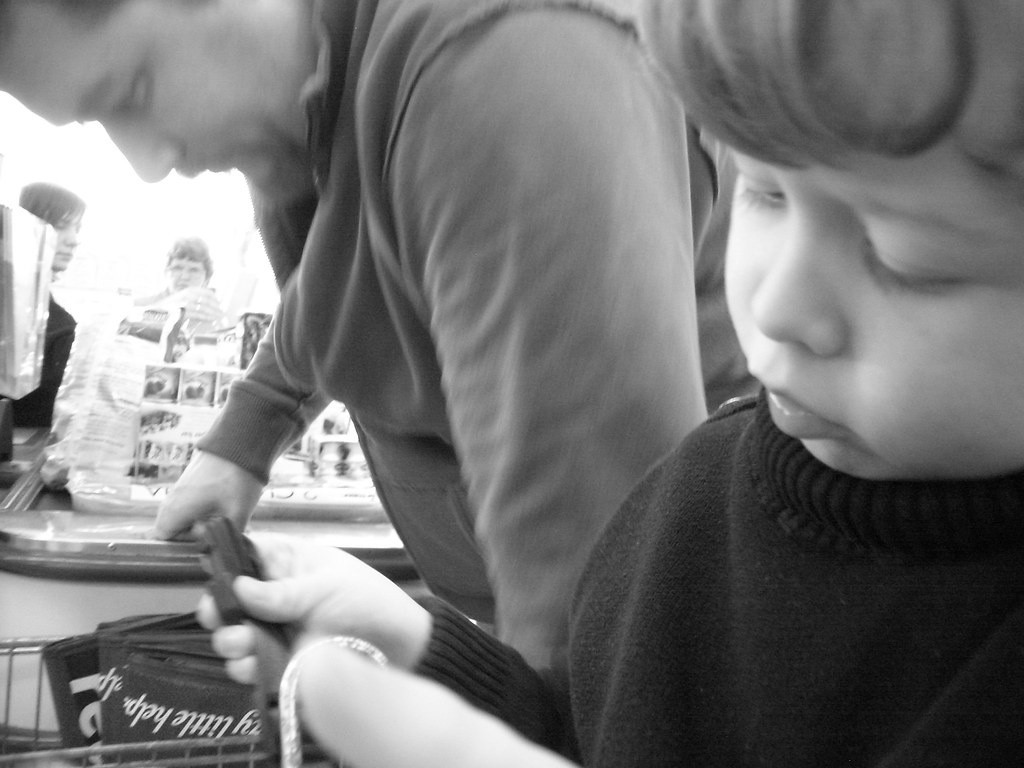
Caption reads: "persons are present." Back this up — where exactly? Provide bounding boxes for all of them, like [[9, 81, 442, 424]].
[[0, 0, 767, 672], [200, 0, 1024, 768], [0, 182, 85, 428], [130, 237, 223, 323]]
